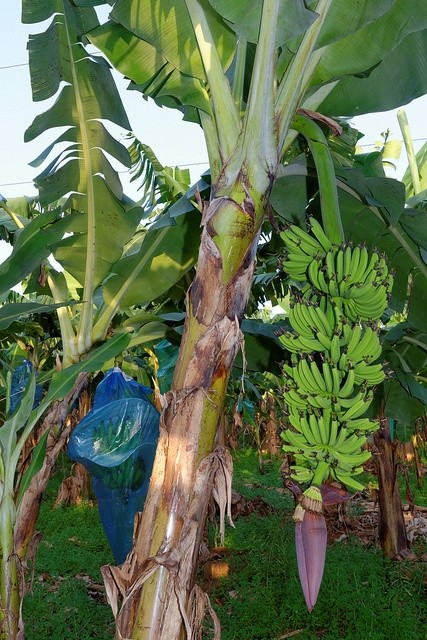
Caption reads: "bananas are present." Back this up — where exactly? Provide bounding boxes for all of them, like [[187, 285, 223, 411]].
[[277, 215, 396, 519], [92, 419, 146, 505]]
